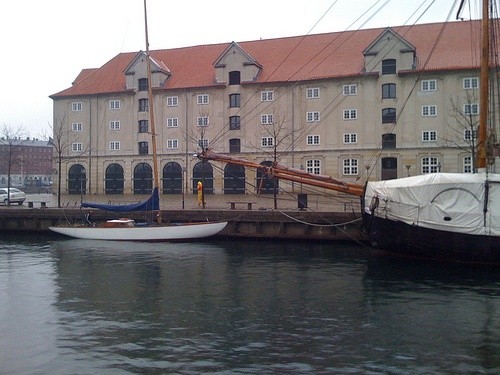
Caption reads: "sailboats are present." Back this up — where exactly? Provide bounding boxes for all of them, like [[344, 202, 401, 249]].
[[192, 0, 500, 267], [48, 0, 234, 243]]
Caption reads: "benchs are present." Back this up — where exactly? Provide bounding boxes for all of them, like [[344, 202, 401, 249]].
[[226, 201, 257, 210], [341, 202, 362, 211], [24, 201, 50, 208]]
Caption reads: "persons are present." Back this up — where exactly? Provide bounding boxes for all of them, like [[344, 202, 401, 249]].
[[197, 182, 206, 204]]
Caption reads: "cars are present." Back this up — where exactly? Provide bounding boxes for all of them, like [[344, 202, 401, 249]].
[[0, 187, 26, 205]]
